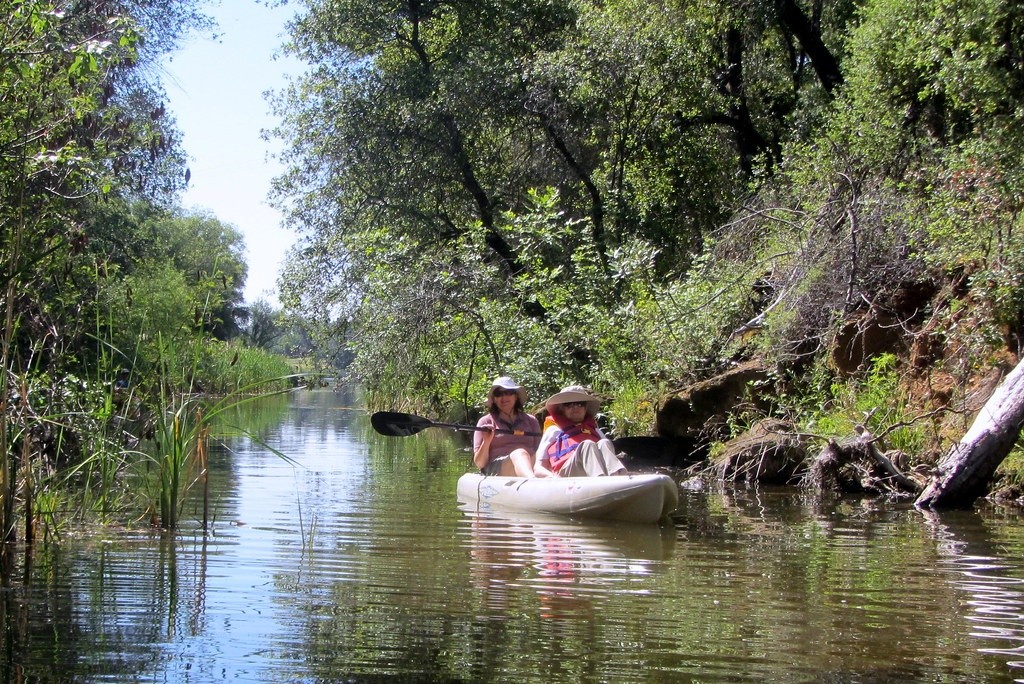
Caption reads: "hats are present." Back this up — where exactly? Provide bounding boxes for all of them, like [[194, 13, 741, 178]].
[[483, 377, 529, 406], [545, 385, 600, 418]]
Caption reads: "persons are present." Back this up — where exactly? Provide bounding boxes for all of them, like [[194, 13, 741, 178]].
[[473, 377, 541, 478], [533, 386, 628, 477]]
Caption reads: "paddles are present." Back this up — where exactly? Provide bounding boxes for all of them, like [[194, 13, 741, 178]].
[[371, 412, 684, 464]]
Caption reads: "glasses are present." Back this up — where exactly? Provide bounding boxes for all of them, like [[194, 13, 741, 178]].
[[493, 389, 516, 396], [564, 401, 587, 408]]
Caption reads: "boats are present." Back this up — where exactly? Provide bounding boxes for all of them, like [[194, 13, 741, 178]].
[[457, 471, 679, 524]]
